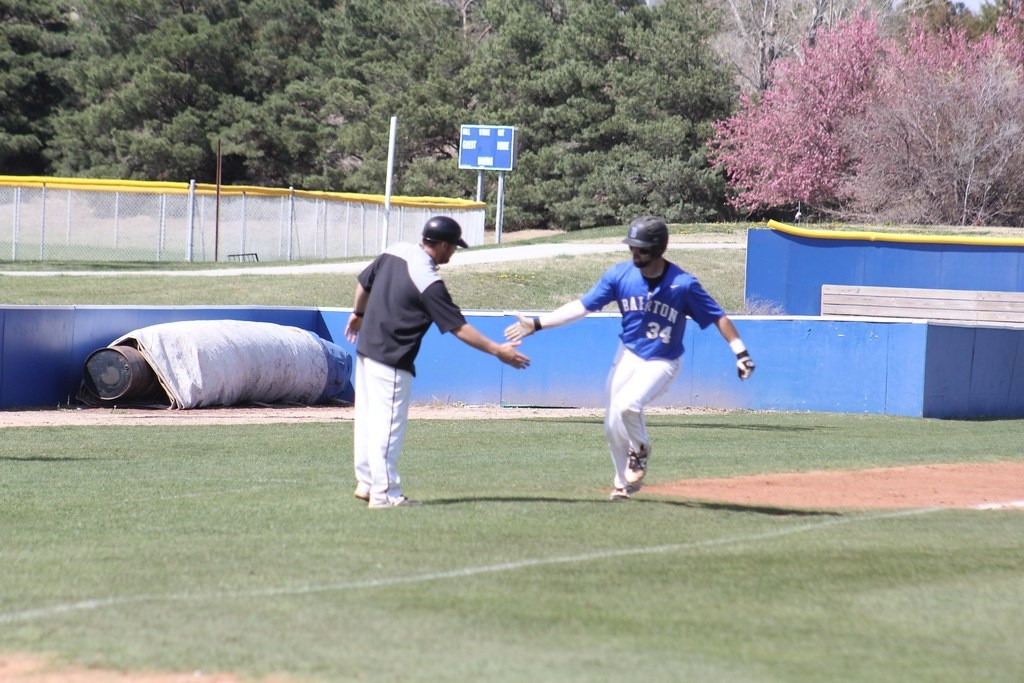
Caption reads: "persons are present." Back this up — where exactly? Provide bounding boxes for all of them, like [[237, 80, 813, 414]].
[[505, 215, 755, 501], [344, 217, 530, 511]]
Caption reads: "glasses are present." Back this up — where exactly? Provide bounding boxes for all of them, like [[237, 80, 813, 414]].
[[629, 246, 654, 255]]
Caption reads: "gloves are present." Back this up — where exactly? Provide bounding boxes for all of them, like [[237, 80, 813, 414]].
[[736, 349, 755, 381]]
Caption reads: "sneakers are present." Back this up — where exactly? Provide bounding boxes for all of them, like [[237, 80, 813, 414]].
[[625, 442, 652, 485], [354, 481, 407, 501], [368, 494, 424, 506], [610, 482, 641, 499]]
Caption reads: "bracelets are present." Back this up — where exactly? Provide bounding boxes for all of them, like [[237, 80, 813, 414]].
[[534, 316, 542, 331], [353, 310, 364, 318], [736, 350, 749, 359]]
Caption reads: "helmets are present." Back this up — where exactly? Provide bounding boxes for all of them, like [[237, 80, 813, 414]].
[[621, 216, 668, 259], [422, 216, 469, 248]]
